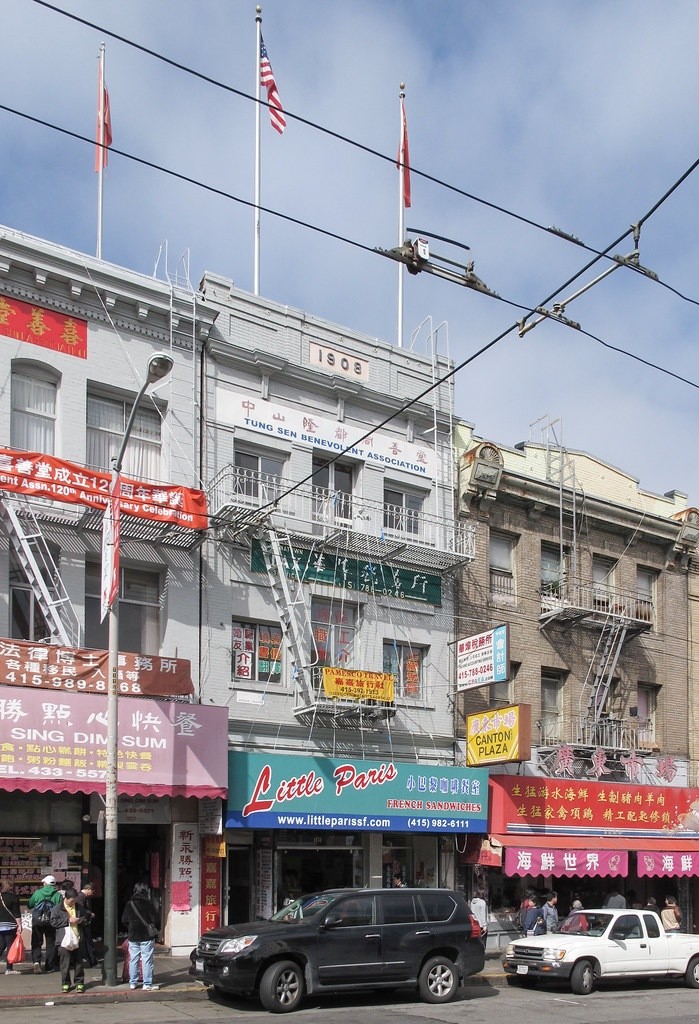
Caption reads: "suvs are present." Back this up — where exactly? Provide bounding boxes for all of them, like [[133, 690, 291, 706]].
[[189, 889, 487, 1013]]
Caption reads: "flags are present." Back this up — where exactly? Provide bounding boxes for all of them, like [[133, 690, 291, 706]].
[[397, 105, 411, 207], [95, 65, 112, 172], [260, 34, 287, 134]]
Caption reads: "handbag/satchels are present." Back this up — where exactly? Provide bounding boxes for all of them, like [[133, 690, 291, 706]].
[[526, 930, 534, 937], [147, 924, 159, 938], [61, 926, 79, 950], [7, 932, 26, 965]]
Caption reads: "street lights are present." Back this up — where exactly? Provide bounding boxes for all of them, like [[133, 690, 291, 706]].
[[103, 351, 175, 988]]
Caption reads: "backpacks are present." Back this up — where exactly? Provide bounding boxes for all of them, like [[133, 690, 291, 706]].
[[32, 889, 57, 925]]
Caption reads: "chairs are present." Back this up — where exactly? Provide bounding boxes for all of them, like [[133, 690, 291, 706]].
[[624, 926, 640, 938], [341, 902, 360, 927]]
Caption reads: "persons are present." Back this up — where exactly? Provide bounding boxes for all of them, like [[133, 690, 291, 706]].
[[394, 874, 407, 888], [27, 876, 103, 973], [601, 884, 683, 933], [0, 878, 23, 975], [520, 886, 558, 937], [51, 889, 88, 992], [122, 885, 161, 991], [471, 887, 489, 946], [570, 892, 589, 931]]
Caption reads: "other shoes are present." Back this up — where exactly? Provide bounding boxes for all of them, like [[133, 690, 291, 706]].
[[34, 962, 42, 974], [46, 969, 55, 973], [5, 969, 22, 975]]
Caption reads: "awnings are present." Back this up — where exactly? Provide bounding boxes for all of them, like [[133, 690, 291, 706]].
[[489, 833, 699, 878]]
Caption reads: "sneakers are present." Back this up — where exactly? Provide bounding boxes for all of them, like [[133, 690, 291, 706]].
[[130, 984, 137, 990], [61, 985, 70, 993], [142, 983, 159, 991], [76, 983, 85, 993]]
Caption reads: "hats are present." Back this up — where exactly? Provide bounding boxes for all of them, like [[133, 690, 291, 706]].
[[42, 875, 56, 887]]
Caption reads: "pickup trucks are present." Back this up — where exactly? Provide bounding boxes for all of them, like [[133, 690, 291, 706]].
[[502, 908, 699, 995]]
